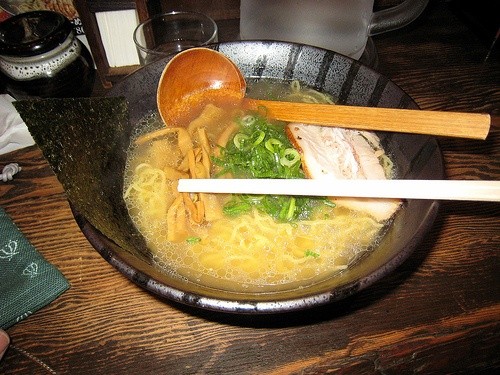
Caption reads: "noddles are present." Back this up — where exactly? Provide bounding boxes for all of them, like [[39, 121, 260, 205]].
[[121, 79, 401, 285]]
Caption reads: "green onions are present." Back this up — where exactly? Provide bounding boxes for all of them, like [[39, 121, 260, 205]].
[[211, 103, 336, 224]]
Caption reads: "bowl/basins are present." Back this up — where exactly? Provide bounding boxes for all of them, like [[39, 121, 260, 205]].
[[65, 40, 446, 315]]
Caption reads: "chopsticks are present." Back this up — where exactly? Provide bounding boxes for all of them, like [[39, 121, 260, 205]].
[[178, 178, 500, 202]]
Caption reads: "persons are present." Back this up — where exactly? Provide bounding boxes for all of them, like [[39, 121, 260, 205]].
[[0, 330, 10, 360]]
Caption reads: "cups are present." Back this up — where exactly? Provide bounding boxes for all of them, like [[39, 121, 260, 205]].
[[240, 0, 427, 63], [133, 10, 218, 67]]
